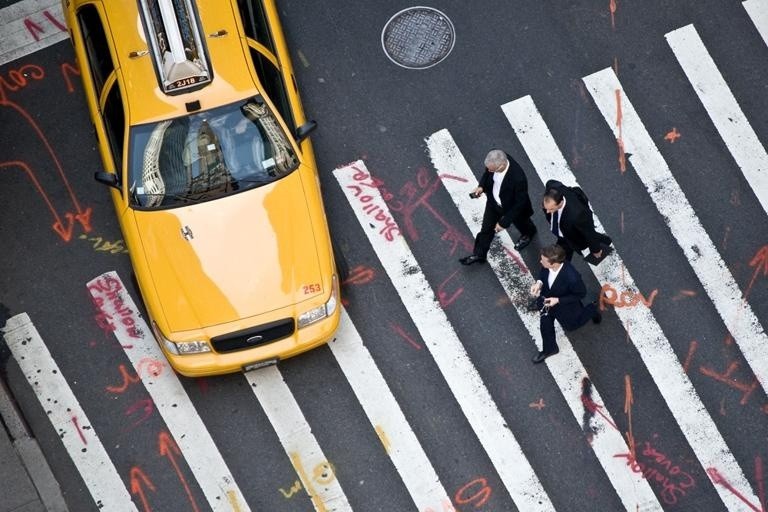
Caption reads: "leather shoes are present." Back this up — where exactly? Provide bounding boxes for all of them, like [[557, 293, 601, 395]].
[[533, 348, 559, 364], [526, 298, 544, 310], [514, 232, 533, 250], [458, 253, 487, 266], [589, 304, 602, 325]]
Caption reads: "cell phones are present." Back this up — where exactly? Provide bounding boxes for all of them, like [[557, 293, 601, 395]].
[[469, 192, 480, 199]]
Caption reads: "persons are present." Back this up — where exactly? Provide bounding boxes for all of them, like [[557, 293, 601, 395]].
[[459, 148, 536, 271], [229, 112, 260, 135], [529, 244, 601, 364], [540, 178, 612, 263]]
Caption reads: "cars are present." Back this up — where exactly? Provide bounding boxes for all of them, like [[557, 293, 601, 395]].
[[61, 1, 342, 379]]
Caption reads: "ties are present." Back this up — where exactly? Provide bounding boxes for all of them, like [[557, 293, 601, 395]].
[[552, 211, 558, 237]]
